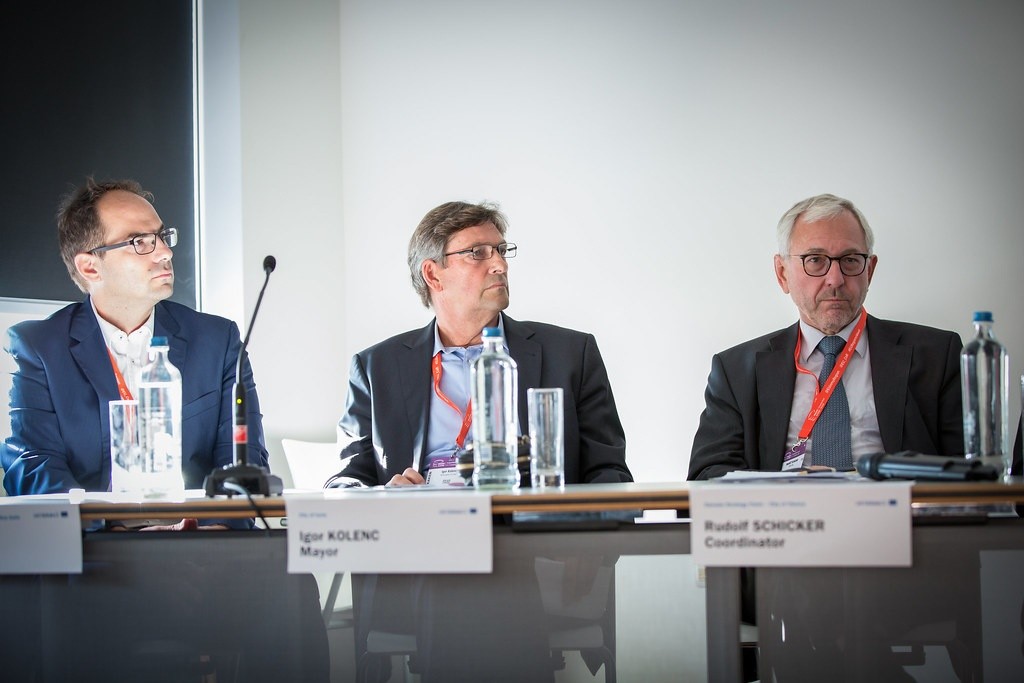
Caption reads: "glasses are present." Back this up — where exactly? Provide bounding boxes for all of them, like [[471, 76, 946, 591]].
[[89, 227, 179, 255], [790, 253, 869, 276], [442, 243, 517, 260]]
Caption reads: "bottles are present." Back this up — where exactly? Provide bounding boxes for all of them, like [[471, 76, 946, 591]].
[[960, 310, 1008, 475], [136, 337, 184, 503], [470, 328, 519, 487]]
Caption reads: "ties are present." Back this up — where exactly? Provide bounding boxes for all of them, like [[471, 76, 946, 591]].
[[811, 335, 853, 471]]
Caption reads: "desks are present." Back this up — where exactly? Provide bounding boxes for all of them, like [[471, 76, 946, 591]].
[[0, 469, 1024, 683]]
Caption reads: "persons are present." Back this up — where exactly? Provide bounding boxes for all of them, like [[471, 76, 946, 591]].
[[0, 180, 330, 683], [678, 192, 1001, 683], [322, 201, 643, 682]]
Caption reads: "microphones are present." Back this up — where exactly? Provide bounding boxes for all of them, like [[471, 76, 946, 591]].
[[203, 255, 283, 498], [856, 450, 1000, 483]]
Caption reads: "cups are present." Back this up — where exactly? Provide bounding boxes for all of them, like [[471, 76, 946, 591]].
[[110, 400, 140, 501], [527, 388, 564, 487]]
